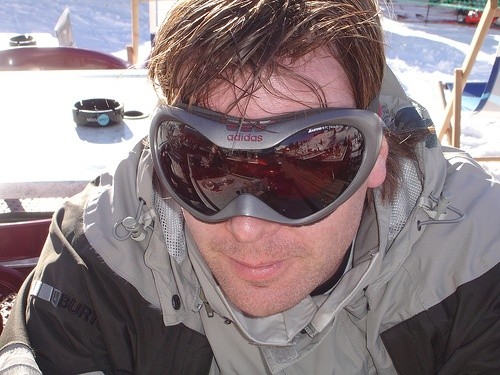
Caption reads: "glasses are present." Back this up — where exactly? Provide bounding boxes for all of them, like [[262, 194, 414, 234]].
[[149, 95, 383, 227]]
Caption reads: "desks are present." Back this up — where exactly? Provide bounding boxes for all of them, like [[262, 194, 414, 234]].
[[0, 33, 57, 52], [0, 69, 168, 200]]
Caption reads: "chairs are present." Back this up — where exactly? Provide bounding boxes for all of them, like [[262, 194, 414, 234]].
[[55, 6, 77, 49], [437, 0, 500, 162]]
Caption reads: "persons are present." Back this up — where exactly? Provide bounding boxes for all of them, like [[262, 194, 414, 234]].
[[209, 147, 227, 178], [181, 136, 193, 154], [344, 134, 353, 161], [0, 0, 500, 375]]
